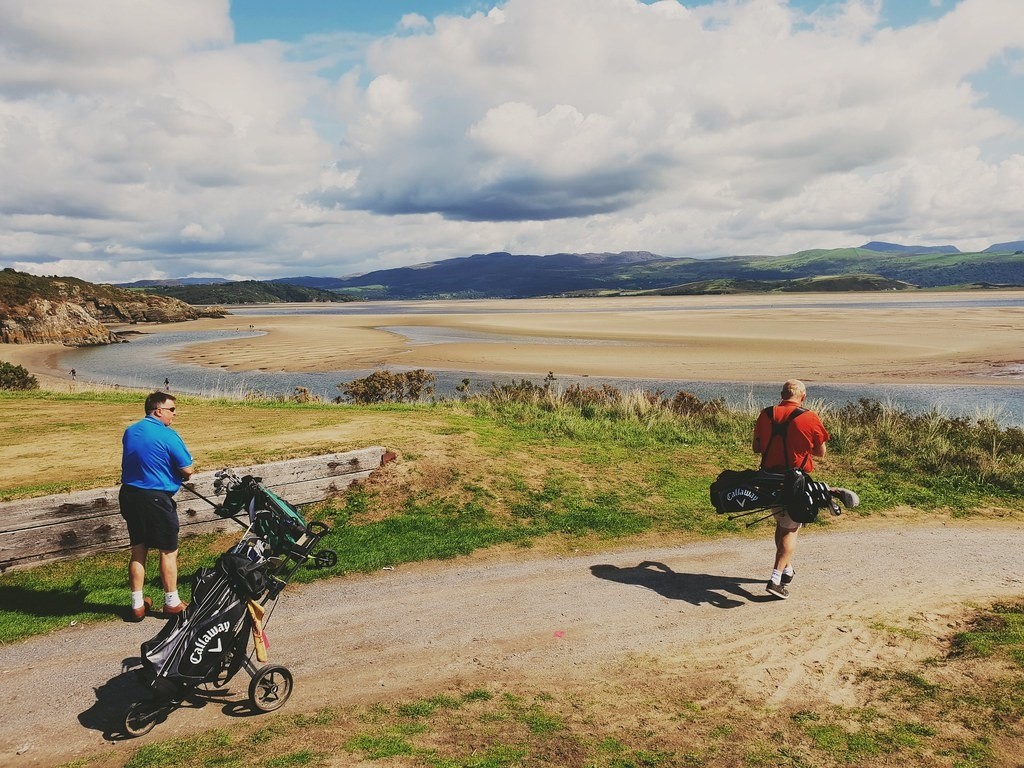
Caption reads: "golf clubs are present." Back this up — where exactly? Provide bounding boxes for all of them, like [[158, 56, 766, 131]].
[[230, 509, 271, 553], [728, 486, 860, 527], [213, 465, 244, 493]]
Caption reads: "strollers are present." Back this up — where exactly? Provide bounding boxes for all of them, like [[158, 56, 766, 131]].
[[122, 476, 330, 738]]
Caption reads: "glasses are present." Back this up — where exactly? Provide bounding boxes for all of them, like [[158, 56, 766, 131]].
[[158, 407, 175, 412]]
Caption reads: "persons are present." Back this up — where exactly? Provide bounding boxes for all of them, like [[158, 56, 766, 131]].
[[752, 380, 829, 599], [69, 368, 76, 380], [119, 391, 193, 623], [164, 378, 170, 390]]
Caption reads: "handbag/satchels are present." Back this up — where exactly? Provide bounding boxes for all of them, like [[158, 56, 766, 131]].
[[786, 468, 819, 523]]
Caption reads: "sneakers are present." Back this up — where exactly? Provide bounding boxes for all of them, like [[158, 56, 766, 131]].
[[765, 580, 791, 598], [782, 569, 795, 585]]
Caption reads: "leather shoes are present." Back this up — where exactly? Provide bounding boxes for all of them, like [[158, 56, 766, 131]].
[[163, 600, 188, 616], [131, 597, 153, 621]]
